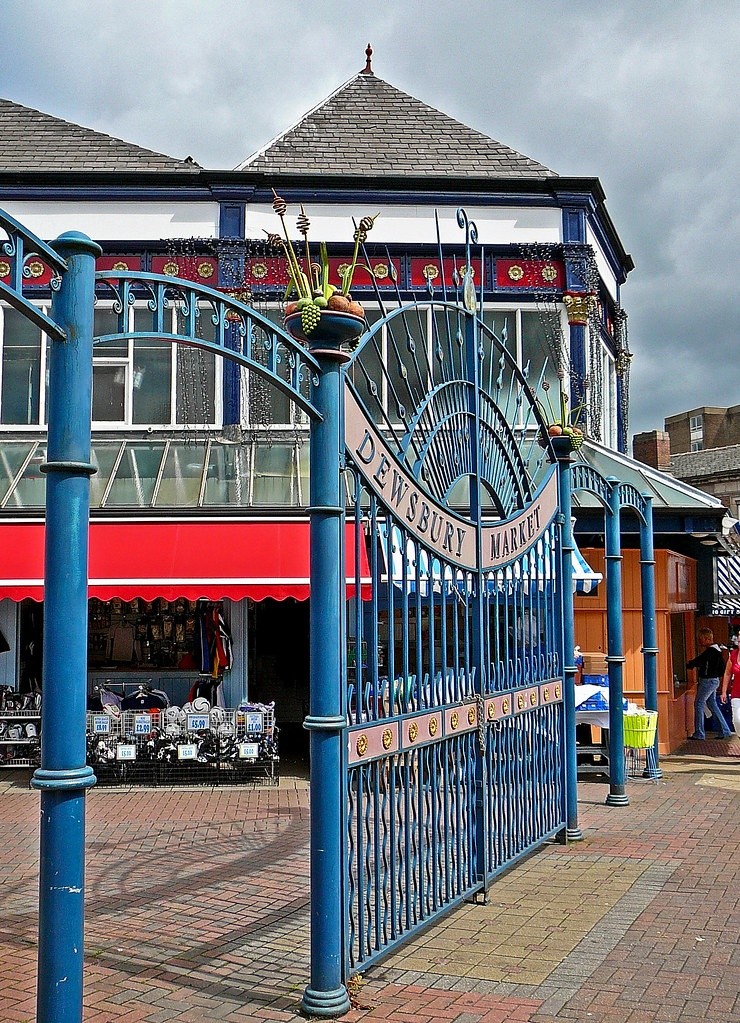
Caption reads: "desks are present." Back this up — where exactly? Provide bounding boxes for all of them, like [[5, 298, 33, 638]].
[[577, 711, 611, 779]]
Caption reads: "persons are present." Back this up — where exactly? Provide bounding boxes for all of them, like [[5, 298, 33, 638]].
[[686, 627, 740, 740]]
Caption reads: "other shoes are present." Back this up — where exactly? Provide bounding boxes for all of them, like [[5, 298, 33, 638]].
[[687, 736, 704, 740], [714, 735, 731, 740]]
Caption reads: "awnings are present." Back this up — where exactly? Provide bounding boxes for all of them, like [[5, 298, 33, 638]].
[[377, 524, 603, 595], [0, 524, 374, 604]]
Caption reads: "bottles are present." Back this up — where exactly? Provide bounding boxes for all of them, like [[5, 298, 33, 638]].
[[674, 675, 679, 687], [729, 626, 734, 642], [704, 705, 712, 718]]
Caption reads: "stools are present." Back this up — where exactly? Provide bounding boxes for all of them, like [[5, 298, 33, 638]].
[[624, 745, 658, 786]]
